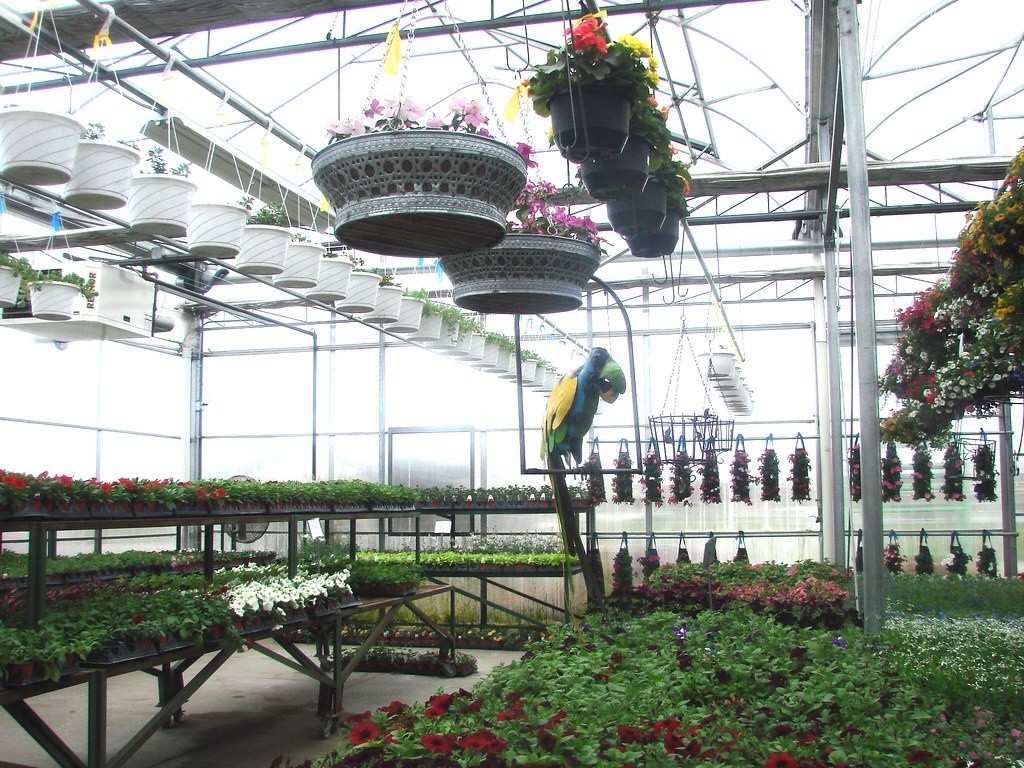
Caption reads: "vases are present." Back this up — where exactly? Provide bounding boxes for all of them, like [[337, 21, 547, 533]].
[[436, 233, 601, 316], [0, 101, 86, 188], [579, 99, 694, 258], [311, 132, 528, 260], [544, 81, 637, 164]]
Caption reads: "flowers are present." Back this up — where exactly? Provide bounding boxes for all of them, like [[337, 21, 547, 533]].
[[326, 91, 539, 174], [516, 9, 660, 118], [0, 467, 589, 694], [583, 434, 1001, 601], [872, 145, 1024, 445], [501, 175, 610, 257]]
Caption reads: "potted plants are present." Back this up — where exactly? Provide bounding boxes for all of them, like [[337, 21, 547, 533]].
[[62, 121, 143, 209], [185, 195, 255, 260], [272, 231, 327, 289], [302, 251, 366, 303], [0, 252, 36, 309], [358, 274, 406, 324], [332, 265, 383, 314], [28, 270, 100, 322], [126, 145, 200, 236], [385, 290, 567, 401], [234, 198, 292, 275]]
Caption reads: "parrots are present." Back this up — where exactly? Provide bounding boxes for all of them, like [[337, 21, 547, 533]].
[[540, 346, 627, 478]]
